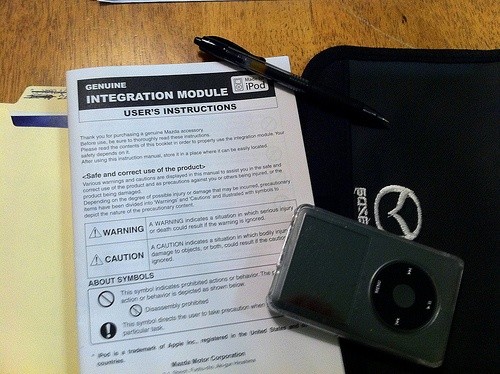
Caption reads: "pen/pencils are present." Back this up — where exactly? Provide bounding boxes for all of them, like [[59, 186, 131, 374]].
[[193, 35, 396, 131]]
